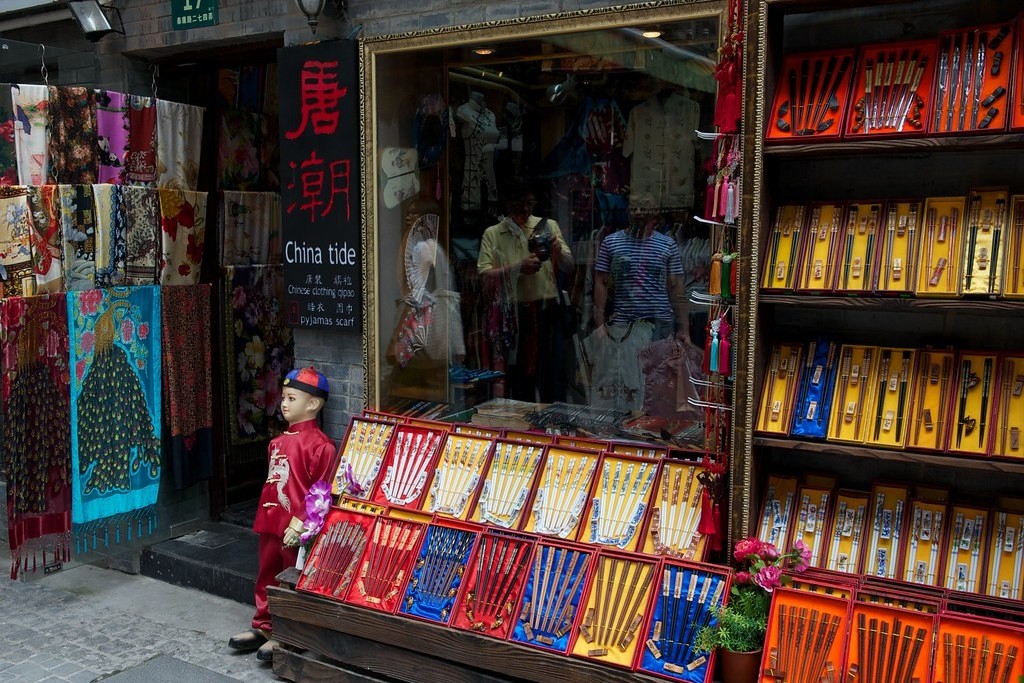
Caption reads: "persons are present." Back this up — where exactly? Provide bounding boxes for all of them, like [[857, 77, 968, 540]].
[[594, 190, 691, 346], [476, 185, 574, 404], [455, 92, 499, 206], [405, 202, 473, 363], [229, 365, 337, 662]]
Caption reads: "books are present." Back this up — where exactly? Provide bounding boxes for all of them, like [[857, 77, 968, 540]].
[[471, 398, 542, 431]]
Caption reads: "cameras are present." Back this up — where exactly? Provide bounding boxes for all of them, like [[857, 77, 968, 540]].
[[528, 236, 553, 262]]
